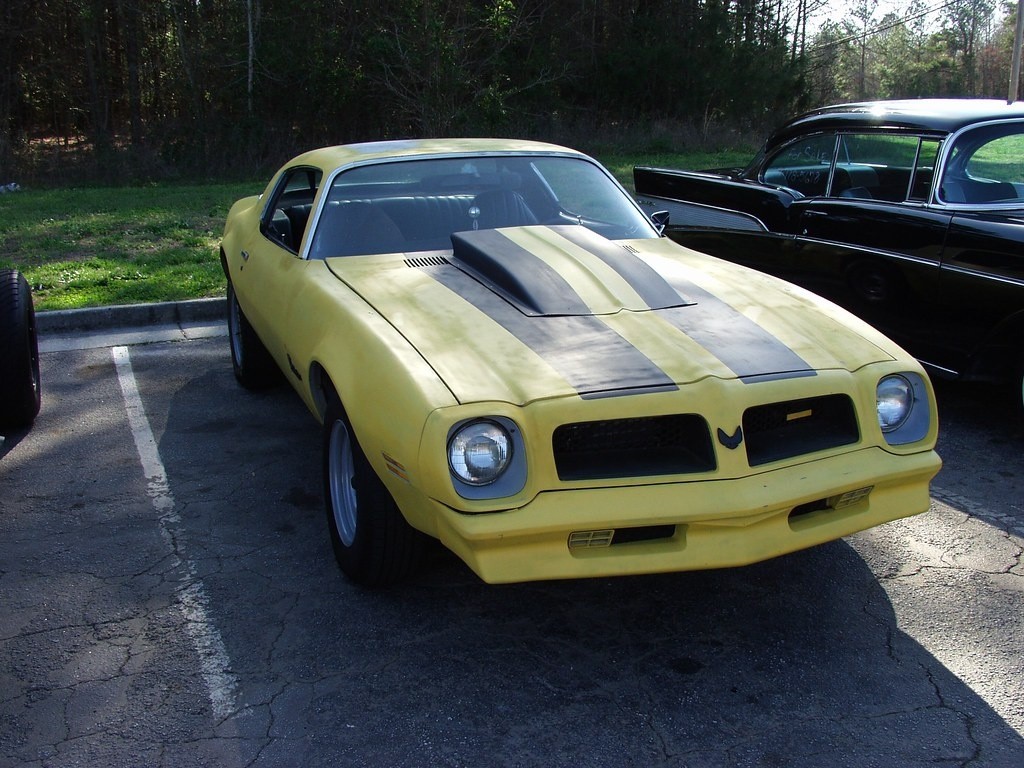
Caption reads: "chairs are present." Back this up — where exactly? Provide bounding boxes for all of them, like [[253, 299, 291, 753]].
[[342, 206, 407, 248], [469, 190, 539, 229], [836, 166, 907, 198]]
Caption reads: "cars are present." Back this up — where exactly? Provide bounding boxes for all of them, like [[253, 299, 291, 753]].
[[633, 99, 1024, 418], [218, 137, 943, 600]]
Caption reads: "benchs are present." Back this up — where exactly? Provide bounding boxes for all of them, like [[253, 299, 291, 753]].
[[945, 182, 1018, 203], [291, 195, 475, 244]]
